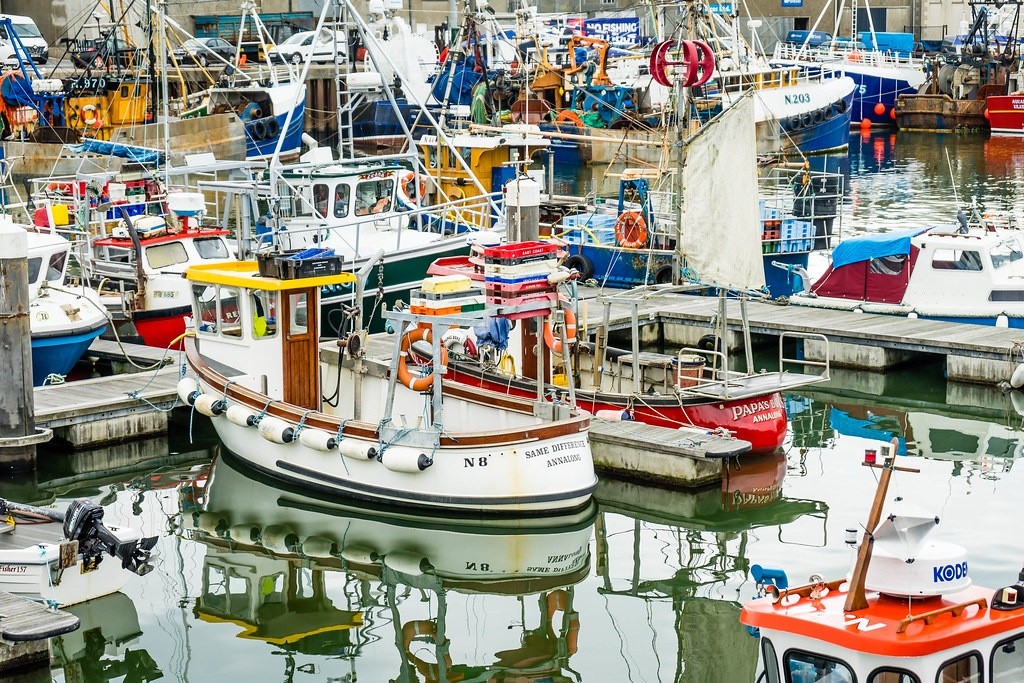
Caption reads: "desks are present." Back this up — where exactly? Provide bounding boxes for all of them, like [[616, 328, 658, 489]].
[[617, 351, 673, 393]]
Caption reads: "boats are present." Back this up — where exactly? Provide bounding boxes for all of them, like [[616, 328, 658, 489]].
[[1, 516, 140, 674], [594, 449, 829, 531], [177, 247, 600, 540], [380, 49, 832, 457], [1, 0, 1024, 396], [163, 438, 593, 683], [737, 435, 1024, 683]]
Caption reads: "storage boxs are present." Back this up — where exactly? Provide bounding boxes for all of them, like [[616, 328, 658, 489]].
[[563, 213, 616, 245], [759, 199, 816, 252], [409, 238, 558, 329], [256, 251, 343, 278]]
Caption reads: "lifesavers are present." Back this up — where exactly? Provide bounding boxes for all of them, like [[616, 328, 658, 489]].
[[402, 620, 453, 680], [614, 211, 647, 249], [45, 183, 70, 193], [546, 590, 581, 656], [397, 327, 449, 392], [823, 106, 833, 120], [813, 109, 823, 125], [251, 122, 266, 140], [802, 113, 813, 128], [265, 119, 279, 139], [80, 104, 98, 125], [654, 262, 673, 284], [401, 173, 426, 203], [543, 306, 576, 353], [564, 254, 595, 283], [836, 100, 846, 113], [787, 116, 801, 131], [441, 329, 477, 355], [697, 334, 721, 352]]
